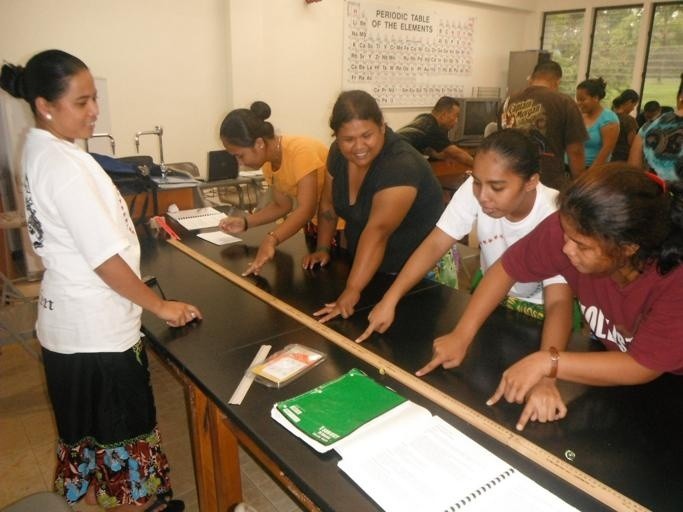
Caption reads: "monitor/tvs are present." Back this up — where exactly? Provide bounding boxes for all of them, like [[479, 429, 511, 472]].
[[448, 97, 502, 146]]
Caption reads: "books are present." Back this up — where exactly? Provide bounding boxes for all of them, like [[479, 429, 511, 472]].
[[336, 414, 580, 511], [166, 207, 228, 231], [270, 368, 412, 454]]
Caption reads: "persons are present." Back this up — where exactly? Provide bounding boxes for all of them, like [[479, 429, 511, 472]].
[[416, 160, 683, 406], [0, 49, 203, 512], [218, 101, 345, 277], [353, 127, 582, 431], [497, 51, 683, 187], [396, 97, 474, 167], [303, 90, 458, 323]]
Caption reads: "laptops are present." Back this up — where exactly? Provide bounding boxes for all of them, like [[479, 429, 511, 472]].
[[194, 150, 239, 182]]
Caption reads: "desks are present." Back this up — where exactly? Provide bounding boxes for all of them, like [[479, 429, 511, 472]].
[[113, 210, 683, 511], [142, 153, 278, 211], [426, 139, 495, 197]]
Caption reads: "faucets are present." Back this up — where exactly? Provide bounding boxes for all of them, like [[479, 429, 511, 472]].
[[84, 133, 116, 156], [135, 125, 167, 179]]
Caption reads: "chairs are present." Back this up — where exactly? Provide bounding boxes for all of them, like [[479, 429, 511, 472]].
[[162, 163, 219, 208], [218, 163, 265, 213], [0, 274, 43, 365]]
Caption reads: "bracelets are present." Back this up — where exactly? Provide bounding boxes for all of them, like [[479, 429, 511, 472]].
[[267, 232, 279, 246], [244, 218, 248, 232], [549, 345, 559, 378]]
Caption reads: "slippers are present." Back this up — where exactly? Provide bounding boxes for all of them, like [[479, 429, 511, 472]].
[[144, 499, 185, 512]]
